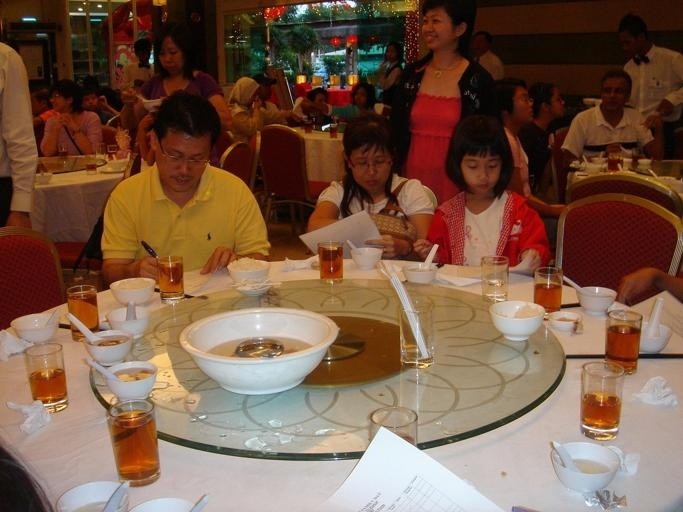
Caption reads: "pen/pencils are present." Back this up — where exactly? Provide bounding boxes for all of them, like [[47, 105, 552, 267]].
[[140, 240, 159, 258]]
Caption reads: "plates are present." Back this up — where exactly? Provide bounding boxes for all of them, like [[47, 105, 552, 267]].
[[99, 167, 124, 173], [236, 286, 271, 296]]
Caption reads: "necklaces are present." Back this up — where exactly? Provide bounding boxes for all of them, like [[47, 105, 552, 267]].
[[430, 58, 461, 79]]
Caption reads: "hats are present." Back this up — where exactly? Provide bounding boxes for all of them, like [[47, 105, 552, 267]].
[[249, 72, 278, 85]]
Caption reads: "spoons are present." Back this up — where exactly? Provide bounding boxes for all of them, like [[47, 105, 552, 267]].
[[126, 300, 137, 321], [134, 93, 146, 101], [86, 358, 119, 382], [236, 338, 285, 361], [64, 313, 104, 344], [645, 298, 664, 337], [562, 275, 592, 297], [420, 244, 439, 270], [600, 152, 603, 159], [582, 154, 589, 165], [190, 495, 209, 512], [553, 442, 581, 472], [617, 163, 623, 172], [100, 481, 129, 512], [45, 308, 60, 328], [648, 169, 659, 180], [40, 170, 44, 176], [347, 240, 356, 249], [620, 144, 627, 152]]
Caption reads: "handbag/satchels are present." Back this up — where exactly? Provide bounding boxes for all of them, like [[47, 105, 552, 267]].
[[369, 177, 418, 243]]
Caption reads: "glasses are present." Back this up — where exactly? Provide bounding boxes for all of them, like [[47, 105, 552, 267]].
[[51, 93, 65, 98], [156, 134, 210, 167], [514, 96, 535, 104], [344, 152, 394, 170]]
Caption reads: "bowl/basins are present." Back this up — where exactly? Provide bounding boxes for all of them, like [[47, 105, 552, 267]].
[[106, 307, 149, 339], [670, 180, 683, 193], [638, 159, 651, 166], [654, 176, 677, 187], [229, 260, 270, 283], [551, 443, 621, 494], [550, 311, 582, 332], [57, 481, 128, 512], [583, 98, 602, 105], [639, 321, 672, 354], [105, 361, 158, 402], [622, 150, 636, 157], [585, 163, 600, 174], [179, 307, 340, 395], [83, 330, 131, 366], [351, 248, 382, 270], [11, 314, 55, 343], [576, 287, 617, 316], [110, 278, 156, 307], [128, 498, 201, 512], [592, 157, 607, 164], [143, 99, 162, 112], [36, 173, 52, 184], [403, 264, 436, 284], [489, 300, 545, 342]]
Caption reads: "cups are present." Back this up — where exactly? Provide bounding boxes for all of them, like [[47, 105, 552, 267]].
[[398, 297, 434, 368], [591, 100, 595, 108], [57, 143, 67, 158], [36, 159, 41, 173], [157, 256, 185, 300], [607, 153, 623, 174], [96, 143, 106, 160], [533, 267, 562, 313], [66, 285, 99, 342], [25, 344, 69, 413], [106, 399, 160, 487], [107, 145, 116, 161], [580, 362, 624, 441], [370, 407, 417, 449], [604, 310, 643, 375], [318, 241, 343, 284], [330, 124, 338, 138], [480, 256, 509, 302], [304, 120, 313, 134], [632, 148, 644, 169], [87, 155, 96, 174]]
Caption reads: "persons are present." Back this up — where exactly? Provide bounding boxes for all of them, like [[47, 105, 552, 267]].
[[518, 80, 565, 198], [561, 70, 663, 164], [617, 13, 683, 161], [377, 42, 402, 92], [228, 77, 301, 222], [80, 89, 118, 124], [0, 41, 39, 230], [120, 38, 155, 105], [471, 32, 505, 79], [40, 78, 103, 155], [119, 24, 233, 171], [303, 80, 377, 123], [615, 267, 682, 305], [306, 88, 338, 129], [495, 78, 566, 219], [251, 72, 287, 128], [101, 91, 270, 284], [309, 117, 434, 259], [388, 0, 503, 205], [413, 116, 550, 276], [30, 88, 60, 130]]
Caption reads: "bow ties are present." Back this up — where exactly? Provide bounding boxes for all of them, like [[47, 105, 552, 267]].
[[631, 51, 653, 66]]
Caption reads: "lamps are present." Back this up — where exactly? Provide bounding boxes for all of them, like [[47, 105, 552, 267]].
[[296, 75, 307, 84], [348, 75, 358, 85], [312, 76, 321, 86], [330, 75, 340, 86]]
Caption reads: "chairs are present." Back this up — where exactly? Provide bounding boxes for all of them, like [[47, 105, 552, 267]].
[[209, 131, 234, 168], [565, 175, 683, 218], [0, 225, 65, 332], [260, 124, 316, 255], [219, 141, 251, 187], [250, 131, 261, 192], [555, 192, 683, 306]]
